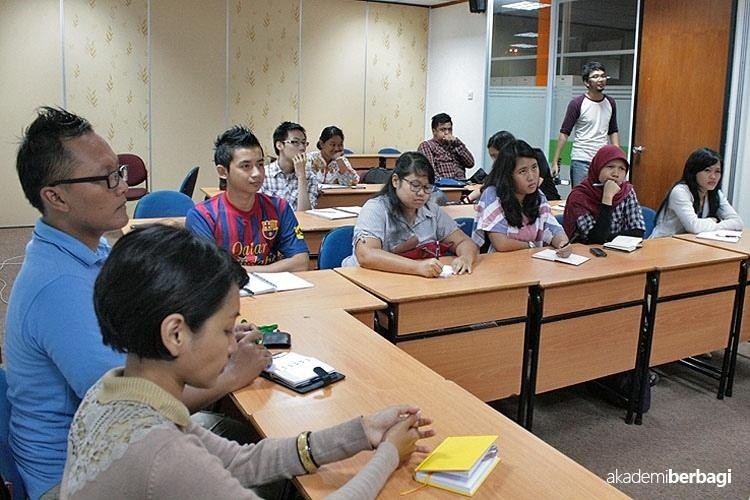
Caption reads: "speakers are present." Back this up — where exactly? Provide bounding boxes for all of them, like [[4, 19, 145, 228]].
[[469, 0, 487, 13]]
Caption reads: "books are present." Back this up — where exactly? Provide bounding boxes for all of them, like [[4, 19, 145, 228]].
[[696, 229, 742, 242], [413, 435, 501, 497], [306, 205, 363, 219], [532, 249, 590, 266], [237, 269, 313, 296], [604, 234, 643, 252], [551, 201, 565, 210], [317, 183, 348, 189]]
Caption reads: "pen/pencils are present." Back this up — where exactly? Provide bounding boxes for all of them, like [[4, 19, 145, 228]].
[[716, 234, 743, 238], [554, 233, 579, 255], [313, 210, 336, 214], [435, 247, 440, 260], [241, 286, 255, 296], [592, 182, 607, 187]]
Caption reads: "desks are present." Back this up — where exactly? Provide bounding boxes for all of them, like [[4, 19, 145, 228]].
[[123, 153, 750, 500]]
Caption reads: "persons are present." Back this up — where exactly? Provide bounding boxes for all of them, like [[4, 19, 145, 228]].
[[257, 120, 320, 212], [550, 62, 621, 190], [5, 103, 274, 500], [62, 222, 436, 500], [418, 113, 475, 179], [563, 144, 661, 387], [644, 148, 744, 358], [185, 124, 311, 273], [304, 125, 361, 184], [456, 131, 561, 205], [471, 139, 573, 258], [352, 152, 480, 277]]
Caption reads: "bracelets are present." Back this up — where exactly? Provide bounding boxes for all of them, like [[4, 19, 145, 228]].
[[462, 196, 471, 204], [296, 427, 319, 474]]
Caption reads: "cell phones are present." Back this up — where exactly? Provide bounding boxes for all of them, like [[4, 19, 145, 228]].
[[590, 248, 608, 258]]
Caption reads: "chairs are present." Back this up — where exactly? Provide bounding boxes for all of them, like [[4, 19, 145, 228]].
[[116, 154, 148, 201], [342, 148, 353, 154], [378, 147, 401, 154]]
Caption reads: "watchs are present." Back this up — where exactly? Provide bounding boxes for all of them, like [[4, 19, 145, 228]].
[[528, 241, 536, 248]]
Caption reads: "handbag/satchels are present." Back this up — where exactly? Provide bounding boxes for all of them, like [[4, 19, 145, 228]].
[[395, 240, 454, 260]]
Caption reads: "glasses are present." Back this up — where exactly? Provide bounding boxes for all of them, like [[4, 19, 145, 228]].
[[49, 164, 129, 189], [585, 73, 608, 79], [400, 177, 436, 194], [282, 140, 310, 146]]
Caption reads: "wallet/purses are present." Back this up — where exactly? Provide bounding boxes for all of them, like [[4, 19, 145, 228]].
[[262, 332, 291, 348]]
